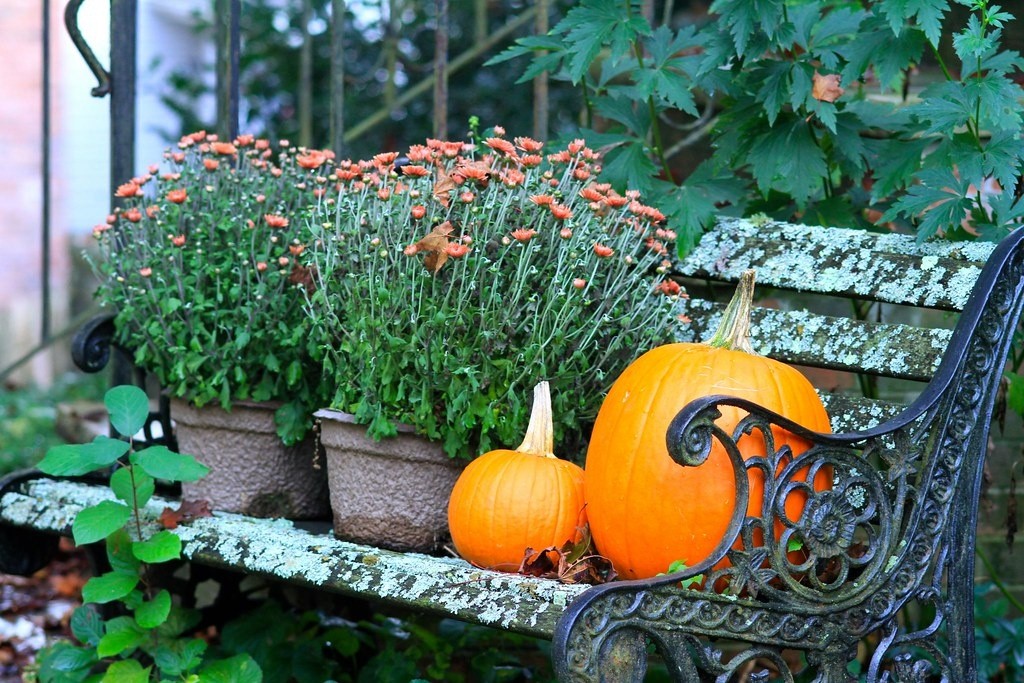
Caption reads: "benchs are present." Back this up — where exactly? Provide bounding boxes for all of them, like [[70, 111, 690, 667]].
[[1, 215, 1024, 683]]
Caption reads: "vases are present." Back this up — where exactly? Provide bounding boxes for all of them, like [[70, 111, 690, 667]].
[[166, 395, 332, 522], [313, 409, 472, 557]]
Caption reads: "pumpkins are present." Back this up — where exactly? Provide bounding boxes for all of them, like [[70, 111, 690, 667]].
[[446, 382, 590, 572], [585, 271, 833, 591]]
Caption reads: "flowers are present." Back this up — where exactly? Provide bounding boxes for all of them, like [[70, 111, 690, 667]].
[[77, 130, 694, 459]]
